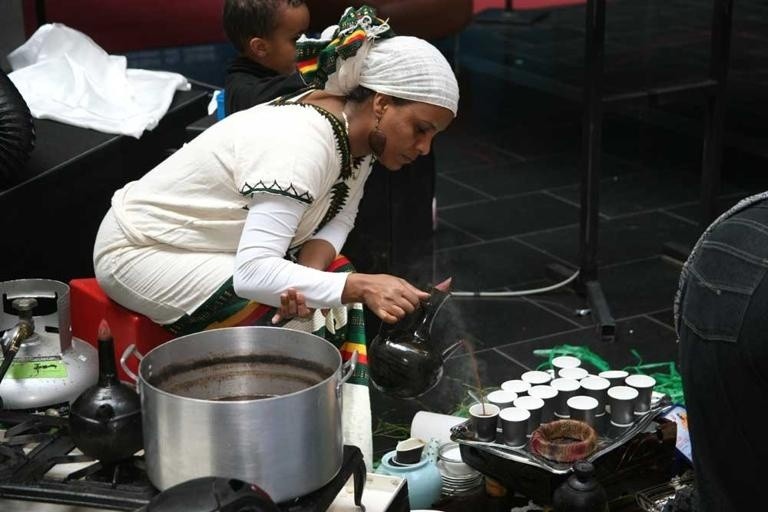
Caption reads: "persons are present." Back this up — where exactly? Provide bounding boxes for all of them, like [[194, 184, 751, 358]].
[[222, 1, 312, 118], [92, 33, 462, 479], [675, 190, 767, 510]]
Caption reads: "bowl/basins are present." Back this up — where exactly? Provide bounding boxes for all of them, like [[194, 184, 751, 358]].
[[438, 442, 472, 475]]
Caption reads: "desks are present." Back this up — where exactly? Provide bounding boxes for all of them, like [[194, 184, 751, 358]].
[[476, 1, 729, 339], [1, 77, 223, 276]]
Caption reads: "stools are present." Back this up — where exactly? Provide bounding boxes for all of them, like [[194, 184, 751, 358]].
[[69, 278, 175, 388]]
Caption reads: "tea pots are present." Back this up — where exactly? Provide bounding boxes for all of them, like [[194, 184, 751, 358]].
[[74, 322, 144, 465], [370, 277, 463, 397]]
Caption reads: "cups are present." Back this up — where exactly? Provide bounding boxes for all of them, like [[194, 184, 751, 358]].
[[599, 370, 630, 409], [580, 376, 611, 417], [469, 356, 589, 449], [566, 394, 599, 426], [625, 374, 656, 416], [607, 385, 639, 427]]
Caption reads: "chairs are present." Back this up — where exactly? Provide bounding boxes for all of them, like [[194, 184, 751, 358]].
[[21, 1, 472, 57]]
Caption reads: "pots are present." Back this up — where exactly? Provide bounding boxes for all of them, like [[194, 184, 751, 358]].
[[120, 327, 358, 502]]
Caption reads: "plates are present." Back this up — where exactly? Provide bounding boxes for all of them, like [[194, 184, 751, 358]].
[[436, 461, 483, 496]]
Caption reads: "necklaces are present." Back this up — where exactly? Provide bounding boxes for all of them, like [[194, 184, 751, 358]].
[[341, 100, 364, 182]]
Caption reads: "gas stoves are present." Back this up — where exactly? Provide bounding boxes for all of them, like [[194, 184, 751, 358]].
[[0, 410, 367, 512]]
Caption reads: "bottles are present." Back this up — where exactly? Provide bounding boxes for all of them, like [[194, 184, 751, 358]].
[[553, 459, 609, 512]]
